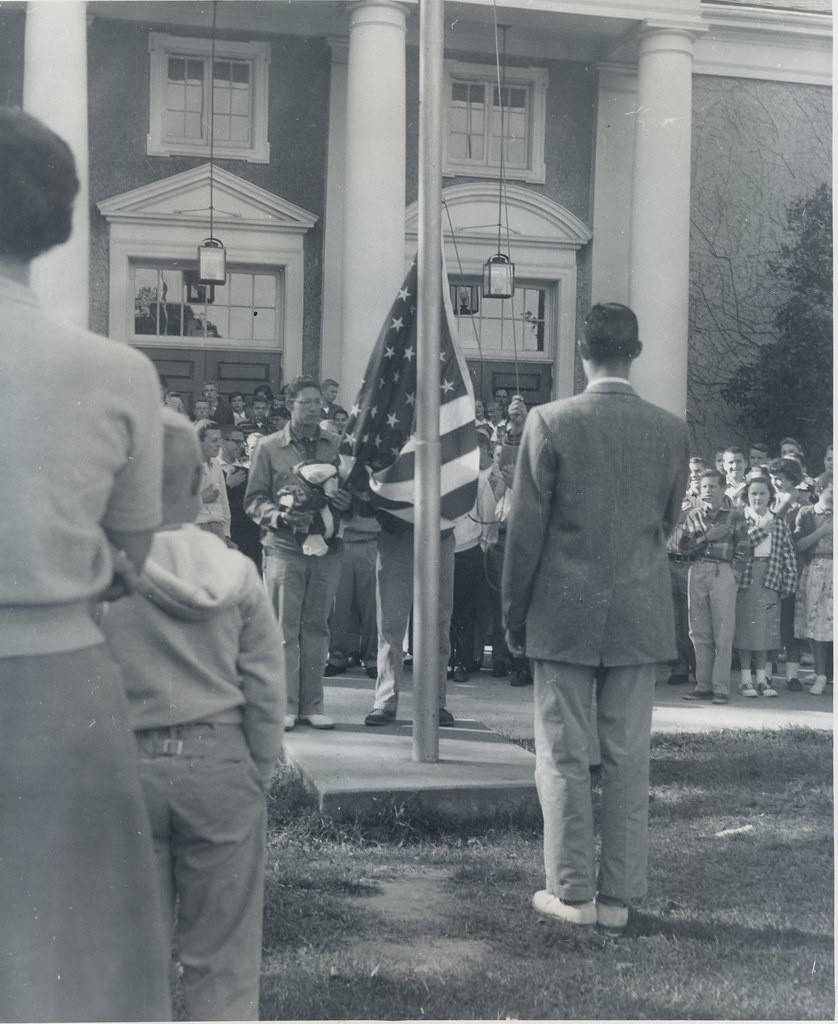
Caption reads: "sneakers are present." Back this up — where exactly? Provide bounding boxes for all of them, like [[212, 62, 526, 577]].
[[533, 889, 598, 926], [594, 900, 628, 934]]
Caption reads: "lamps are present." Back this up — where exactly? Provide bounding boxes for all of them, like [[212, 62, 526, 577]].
[[196, 1, 226, 285], [482, 21, 517, 298]]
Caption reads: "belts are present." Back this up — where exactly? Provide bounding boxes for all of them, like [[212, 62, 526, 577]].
[[668, 553, 687, 562]]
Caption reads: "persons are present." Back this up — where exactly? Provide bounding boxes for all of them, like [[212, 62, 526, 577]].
[[163, 378, 832, 730], [0, 100, 168, 1022], [102, 408, 287, 1021], [501, 301, 689, 928]]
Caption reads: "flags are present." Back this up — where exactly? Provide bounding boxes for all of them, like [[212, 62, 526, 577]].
[[334, 228, 480, 548]]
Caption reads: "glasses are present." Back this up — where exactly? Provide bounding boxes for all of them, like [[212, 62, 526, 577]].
[[289, 399, 323, 409]]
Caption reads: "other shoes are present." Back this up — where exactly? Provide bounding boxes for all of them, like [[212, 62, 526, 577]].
[[492, 668, 508, 677], [712, 693, 727, 703], [800, 652, 814, 664], [810, 676, 826, 695], [445, 658, 481, 682], [365, 708, 396, 725], [758, 676, 777, 697], [805, 673, 817, 684], [667, 674, 688, 684], [511, 666, 532, 686], [786, 678, 801, 691], [284, 713, 297, 729], [298, 713, 335, 729], [438, 709, 454, 725], [322, 650, 359, 677], [738, 682, 758, 697], [366, 667, 377, 678], [683, 691, 712, 700]]
